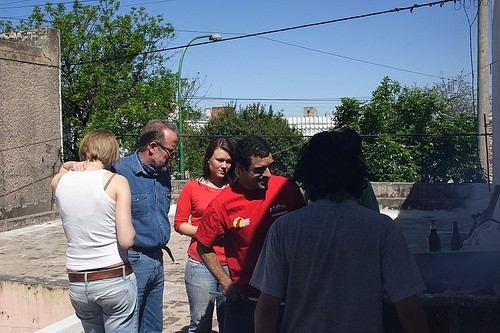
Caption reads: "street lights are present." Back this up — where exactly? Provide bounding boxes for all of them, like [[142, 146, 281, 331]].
[[177, 33, 222, 180]]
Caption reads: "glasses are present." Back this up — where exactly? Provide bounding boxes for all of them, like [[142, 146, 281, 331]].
[[156, 142, 179, 155]]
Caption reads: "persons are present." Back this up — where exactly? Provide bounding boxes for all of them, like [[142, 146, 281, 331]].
[[51, 130, 138, 333], [174, 137, 238, 333], [249, 126, 431, 333], [108, 121, 179, 333], [194, 135, 306, 333]]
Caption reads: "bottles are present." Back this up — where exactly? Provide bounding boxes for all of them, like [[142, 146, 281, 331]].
[[427, 220, 440, 252], [450, 221, 462, 251]]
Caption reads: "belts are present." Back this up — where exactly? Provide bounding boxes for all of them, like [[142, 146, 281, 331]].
[[68, 263, 134, 282]]
